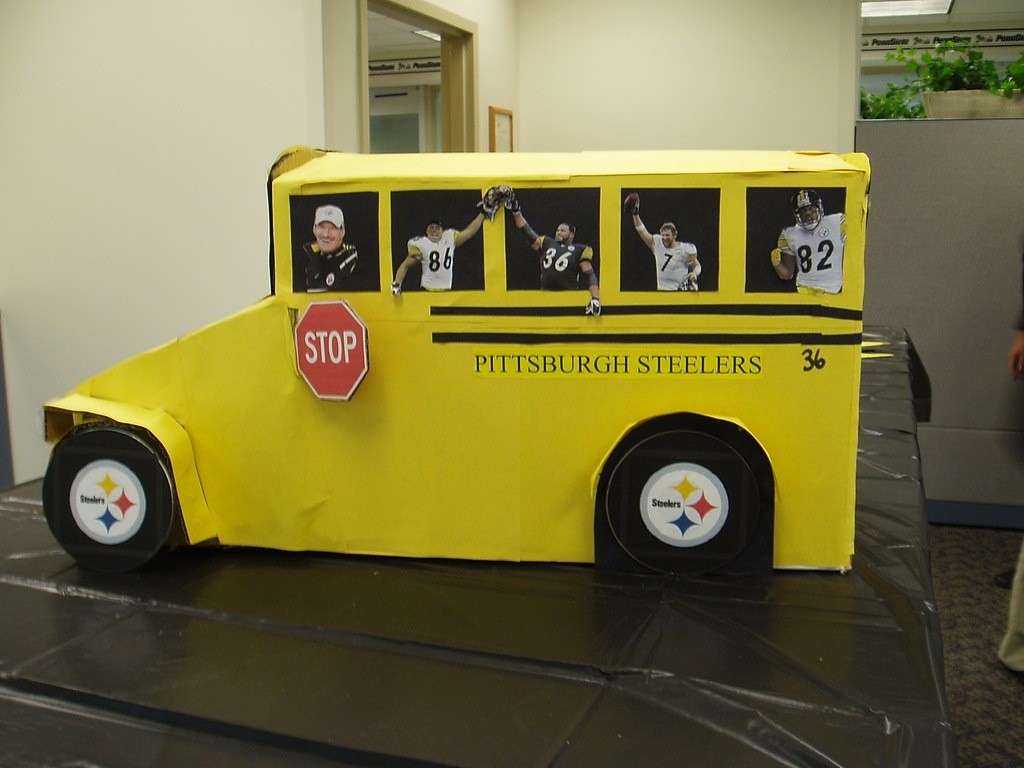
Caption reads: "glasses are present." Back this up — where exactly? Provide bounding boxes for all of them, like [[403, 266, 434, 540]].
[[800, 206, 817, 214]]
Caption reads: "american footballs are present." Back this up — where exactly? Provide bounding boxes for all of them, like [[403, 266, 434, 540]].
[[622, 191, 640, 216]]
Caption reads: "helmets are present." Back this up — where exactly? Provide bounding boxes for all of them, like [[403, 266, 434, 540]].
[[791, 189, 825, 230]]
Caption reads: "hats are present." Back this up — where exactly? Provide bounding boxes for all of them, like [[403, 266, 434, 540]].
[[424, 221, 443, 237], [314, 204, 345, 229]]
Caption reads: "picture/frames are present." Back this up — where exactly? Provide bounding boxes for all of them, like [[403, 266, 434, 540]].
[[488, 104, 513, 152]]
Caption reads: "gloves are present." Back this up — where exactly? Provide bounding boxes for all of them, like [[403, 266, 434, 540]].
[[585, 296, 601, 317], [391, 281, 402, 295]]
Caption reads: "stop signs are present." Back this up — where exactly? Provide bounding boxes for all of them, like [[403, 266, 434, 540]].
[[294, 301, 370, 403]]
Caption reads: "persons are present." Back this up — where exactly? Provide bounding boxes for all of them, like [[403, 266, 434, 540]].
[[498, 184, 602, 317], [292, 206, 361, 293], [997, 228, 1024, 671], [622, 191, 700, 292], [771, 188, 842, 296], [392, 187, 499, 298]]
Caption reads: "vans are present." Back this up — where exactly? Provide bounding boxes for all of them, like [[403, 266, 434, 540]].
[[45, 147, 873, 576]]
